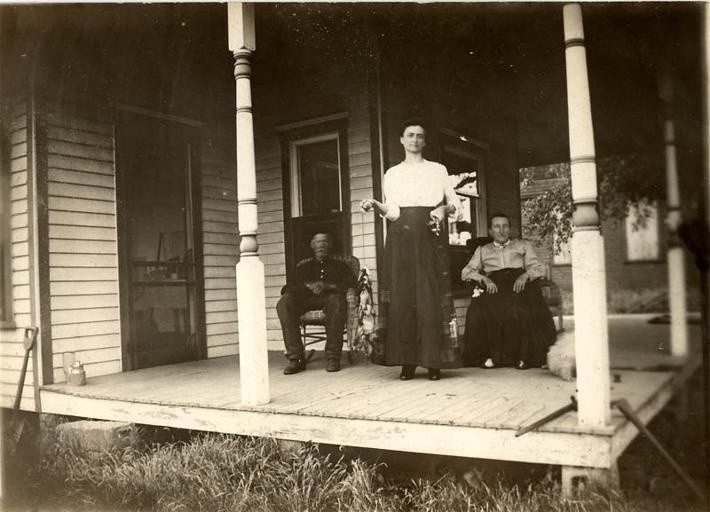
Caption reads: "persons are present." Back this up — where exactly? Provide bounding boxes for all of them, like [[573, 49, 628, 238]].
[[459, 212, 557, 370], [359, 117, 463, 382], [275, 228, 355, 375]]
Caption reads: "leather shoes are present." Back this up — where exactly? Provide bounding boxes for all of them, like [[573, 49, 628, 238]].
[[400, 364, 441, 381], [326, 358, 339, 372], [284, 357, 306, 374]]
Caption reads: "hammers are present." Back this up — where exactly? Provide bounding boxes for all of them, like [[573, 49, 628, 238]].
[[514, 395, 578, 437]]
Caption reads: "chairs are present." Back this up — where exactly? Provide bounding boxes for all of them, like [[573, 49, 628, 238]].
[[281, 255, 375, 372]]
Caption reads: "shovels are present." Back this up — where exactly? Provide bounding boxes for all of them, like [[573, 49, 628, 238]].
[[3, 325, 38, 462]]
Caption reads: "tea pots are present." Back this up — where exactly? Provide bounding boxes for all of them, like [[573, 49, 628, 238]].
[[62, 359, 88, 387]]
